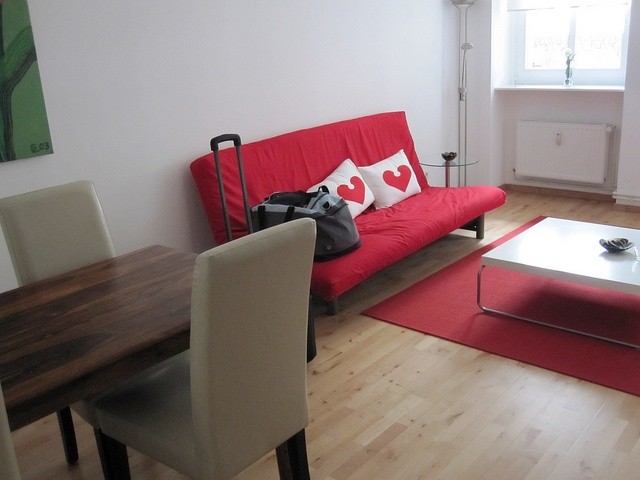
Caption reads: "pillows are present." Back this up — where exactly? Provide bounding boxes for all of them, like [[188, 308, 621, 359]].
[[357, 149, 422, 210], [306, 158, 375, 220]]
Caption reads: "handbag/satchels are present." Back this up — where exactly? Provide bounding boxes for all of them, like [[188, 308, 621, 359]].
[[253, 185, 361, 263]]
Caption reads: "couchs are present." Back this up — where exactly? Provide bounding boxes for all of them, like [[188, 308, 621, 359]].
[[189, 111, 507, 315]]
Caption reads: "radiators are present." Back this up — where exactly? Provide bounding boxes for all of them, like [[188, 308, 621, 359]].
[[513, 120, 611, 186]]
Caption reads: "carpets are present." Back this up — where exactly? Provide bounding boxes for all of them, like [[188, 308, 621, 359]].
[[360, 215, 640, 396]]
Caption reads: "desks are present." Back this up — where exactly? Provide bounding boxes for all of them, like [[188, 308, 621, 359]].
[[1, 244, 199, 432]]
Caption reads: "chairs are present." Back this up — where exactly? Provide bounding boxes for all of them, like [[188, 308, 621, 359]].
[[1, 181, 190, 464], [97, 217, 312, 480]]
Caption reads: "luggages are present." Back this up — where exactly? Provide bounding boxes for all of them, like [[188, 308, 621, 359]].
[[209, 132, 317, 363]]
